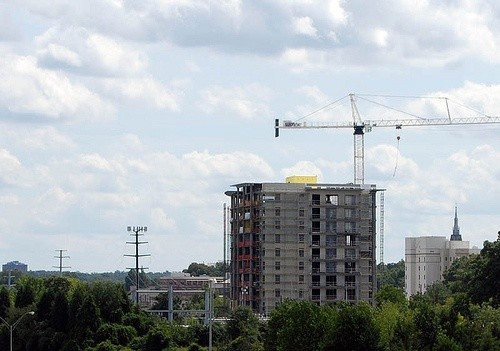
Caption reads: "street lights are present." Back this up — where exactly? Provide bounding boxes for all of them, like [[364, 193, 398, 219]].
[[123, 225, 150, 306], [0, 310, 35, 351]]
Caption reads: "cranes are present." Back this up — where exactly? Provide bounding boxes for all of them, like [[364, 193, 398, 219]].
[[273, 92, 500, 185]]
